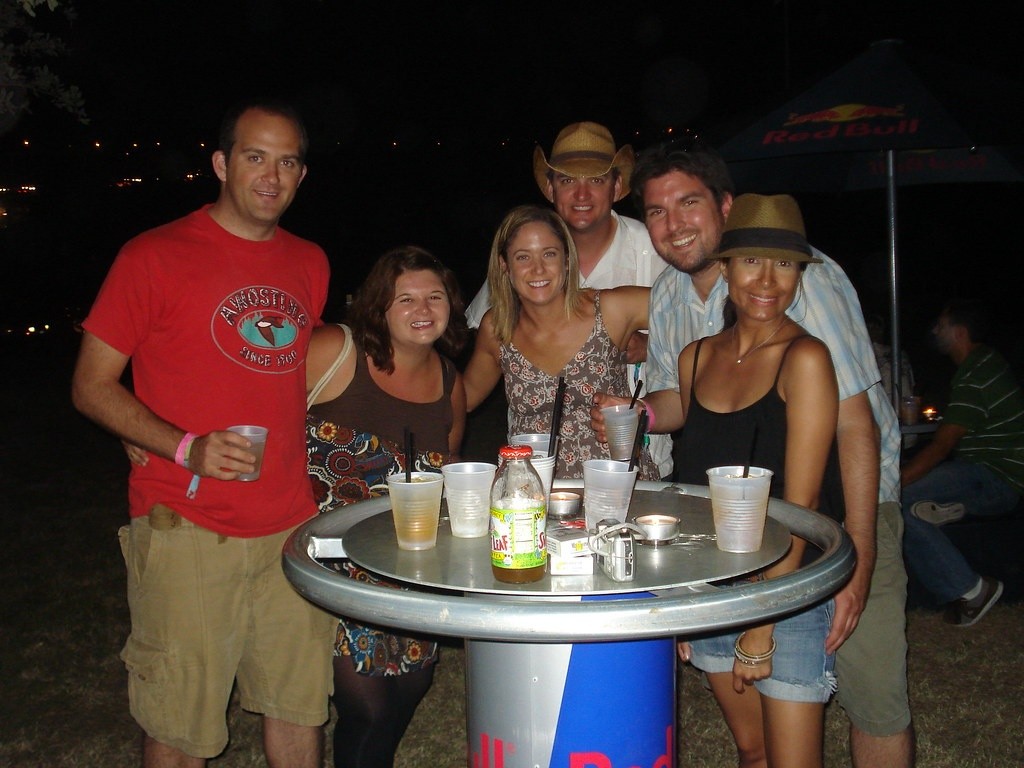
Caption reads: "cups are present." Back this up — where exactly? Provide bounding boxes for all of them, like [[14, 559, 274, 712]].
[[384, 472, 445, 551], [226, 425, 269, 482], [510, 433, 559, 499], [580, 459, 639, 534], [706, 465, 774, 554], [600, 403, 639, 461], [441, 462, 499, 539]]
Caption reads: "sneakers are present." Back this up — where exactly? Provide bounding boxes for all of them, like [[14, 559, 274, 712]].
[[943, 575, 1003, 627]]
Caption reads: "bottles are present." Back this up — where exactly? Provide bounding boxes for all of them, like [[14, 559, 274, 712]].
[[487, 445, 548, 584]]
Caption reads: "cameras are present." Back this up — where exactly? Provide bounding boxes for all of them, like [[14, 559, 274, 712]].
[[595, 518, 636, 583]]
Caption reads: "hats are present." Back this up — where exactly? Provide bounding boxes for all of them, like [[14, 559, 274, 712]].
[[705, 194, 821, 264], [534, 122, 637, 203]]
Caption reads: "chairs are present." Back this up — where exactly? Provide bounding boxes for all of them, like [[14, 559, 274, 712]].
[[940, 494, 1024, 625]]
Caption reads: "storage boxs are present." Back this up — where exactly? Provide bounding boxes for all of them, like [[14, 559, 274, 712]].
[[546, 526, 598, 575]]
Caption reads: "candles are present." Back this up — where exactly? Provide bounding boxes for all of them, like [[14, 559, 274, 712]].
[[548, 492, 581, 517], [634, 514, 680, 540]]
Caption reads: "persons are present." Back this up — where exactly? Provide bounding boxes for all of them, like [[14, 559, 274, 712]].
[[72, 94, 339, 768], [463, 120, 674, 483], [589, 138, 915, 768], [120, 244, 466, 768], [863, 297, 1024, 628], [462, 206, 661, 480], [678, 192, 847, 768]]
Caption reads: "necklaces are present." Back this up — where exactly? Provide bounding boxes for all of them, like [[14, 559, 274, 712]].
[[733, 315, 790, 364]]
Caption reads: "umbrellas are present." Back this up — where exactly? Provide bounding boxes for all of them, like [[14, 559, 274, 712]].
[[712, 40, 1024, 424]]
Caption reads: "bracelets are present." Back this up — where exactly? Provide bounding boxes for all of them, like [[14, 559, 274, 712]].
[[637, 398, 656, 433], [734, 632, 776, 668], [175, 432, 200, 469]]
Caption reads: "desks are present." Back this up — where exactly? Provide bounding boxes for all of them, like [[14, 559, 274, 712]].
[[279, 479, 858, 768]]
